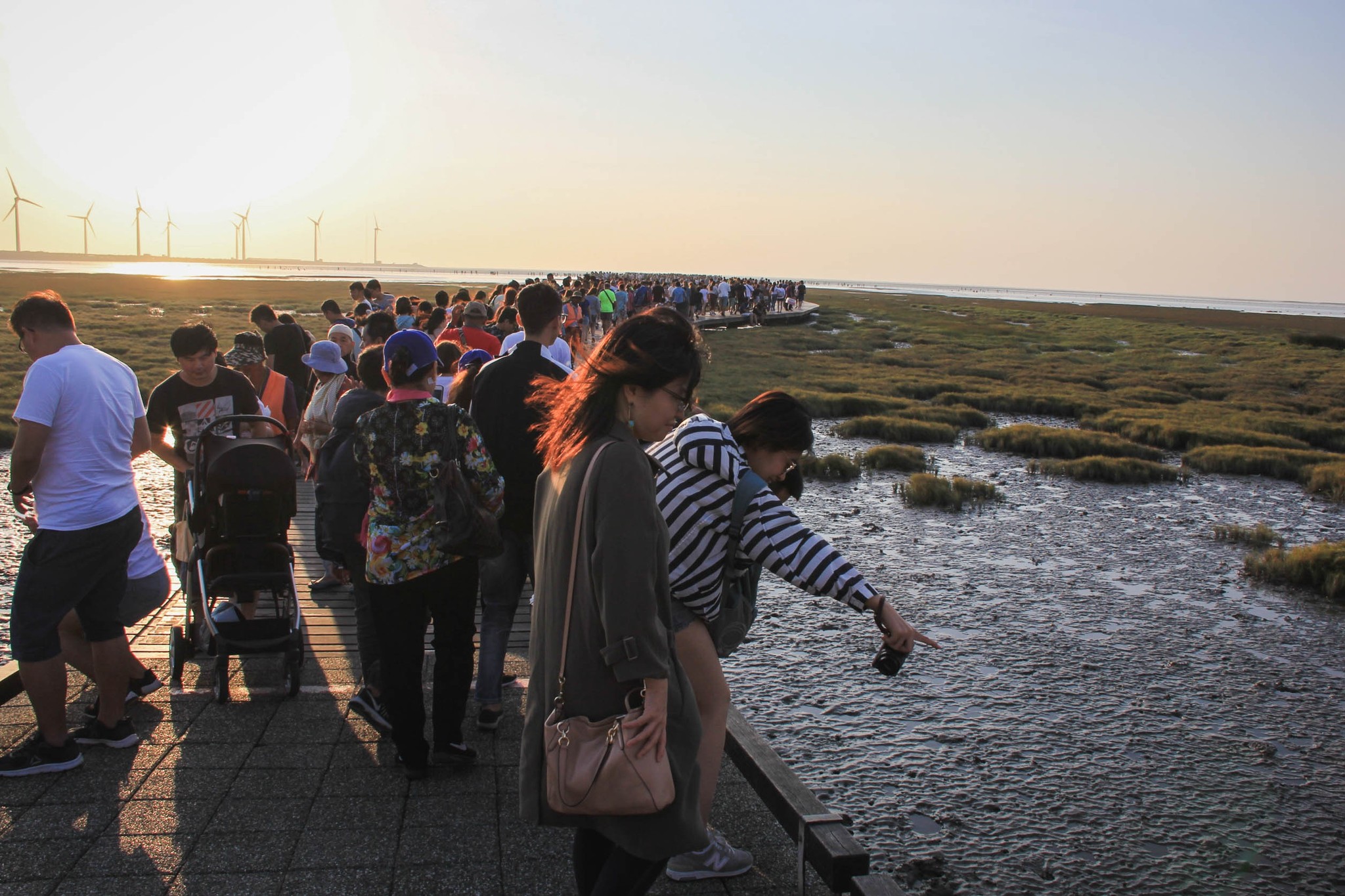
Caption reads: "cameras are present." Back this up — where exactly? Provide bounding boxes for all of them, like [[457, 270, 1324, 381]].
[[870, 632, 911, 676]]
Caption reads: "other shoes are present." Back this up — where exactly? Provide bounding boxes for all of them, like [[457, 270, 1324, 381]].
[[432, 744, 473, 764], [695, 305, 801, 319], [125, 671, 162, 702], [84, 686, 137, 719], [477, 707, 501, 729], [499, 670, 517, 688], [395, 753, 426, 778], [308, 576, 336, 589]]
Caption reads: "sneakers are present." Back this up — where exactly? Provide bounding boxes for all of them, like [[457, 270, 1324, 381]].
[[0, 731, 83, 776], [666, 826, 754, 879], [68, 718, 140, 748], [349, 688, 392, 733]]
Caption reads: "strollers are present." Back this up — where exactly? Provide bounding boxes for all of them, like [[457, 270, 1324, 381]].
[[169, 413, 306, 703]]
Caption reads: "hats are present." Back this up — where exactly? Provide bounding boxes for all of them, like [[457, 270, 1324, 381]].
[[300, 342, 349, 374], [572, 291, 582, 298], [459, 349, 492, 367], [463, 300, 487, 317], [327, 325, 353, 342], [382, 334, 438, 377], [222, 343, 266, 365]]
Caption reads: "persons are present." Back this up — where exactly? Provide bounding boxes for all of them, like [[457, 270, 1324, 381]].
[[211, 267, 816, 601], [352, 328, 509, 773], [145, 322, 277, 621], [644, 388, 944, 881], [0, 290, 171, 776], [519, 305, 711, 896], [459, 279, 579, 731]]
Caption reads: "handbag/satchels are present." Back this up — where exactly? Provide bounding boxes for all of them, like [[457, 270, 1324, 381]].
[[544, 698, 675, 818], [434, 407, 504, 558], [175, 501, 194, 563]]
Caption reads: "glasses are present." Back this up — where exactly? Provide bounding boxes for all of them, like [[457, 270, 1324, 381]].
[[554, 313, 567, 325], [18, 329, 35, 355]]
